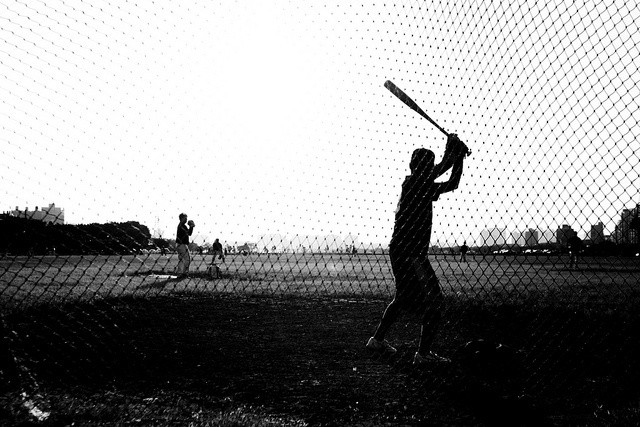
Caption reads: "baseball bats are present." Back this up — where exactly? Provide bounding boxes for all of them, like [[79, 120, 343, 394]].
[[383, 80, 471, 157]]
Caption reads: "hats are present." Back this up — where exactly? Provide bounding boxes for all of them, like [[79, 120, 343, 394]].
[[412, 148, 436, 168]]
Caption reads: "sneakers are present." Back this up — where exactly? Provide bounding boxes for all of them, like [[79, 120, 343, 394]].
[[413, 352, 451, 365], [365, 337, 397, 354]]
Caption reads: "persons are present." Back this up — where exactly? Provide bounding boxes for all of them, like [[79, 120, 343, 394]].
[[568, 232, 582, 268], [176, 213, 195, 278], [211, 238, 224, 264], [366, 133, 468, 365], [459, 241, 468, 262]]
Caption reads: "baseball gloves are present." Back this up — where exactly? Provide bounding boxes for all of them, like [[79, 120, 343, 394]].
[[188, 220, 195, 228]]
